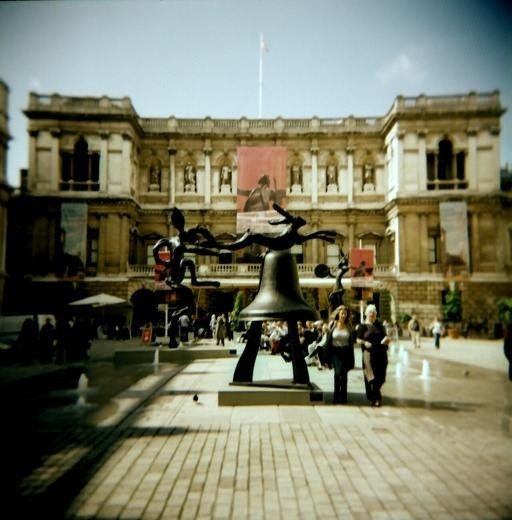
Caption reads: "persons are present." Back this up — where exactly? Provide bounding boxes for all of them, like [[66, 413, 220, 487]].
[[168, 311, 250, 348], [19, 308, 128, 365], [260, 321, 288, 354], [297, 303, 390, 406], [408, 312, 444, 349]]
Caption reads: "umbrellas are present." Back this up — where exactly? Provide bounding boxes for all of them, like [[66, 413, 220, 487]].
[[68, 292, 126, 308]]
